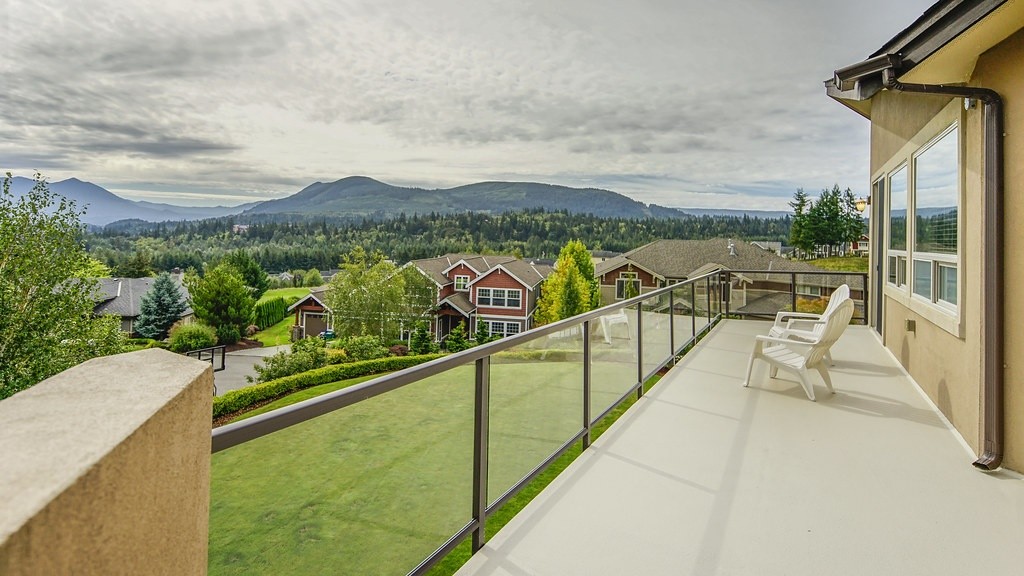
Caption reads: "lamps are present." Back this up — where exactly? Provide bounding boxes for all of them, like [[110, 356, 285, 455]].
[[851, 196, 870, 213]]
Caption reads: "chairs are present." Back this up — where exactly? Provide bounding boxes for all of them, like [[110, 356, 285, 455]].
[[743, 283, 855, 401], [540, 309, 632, 364]]
